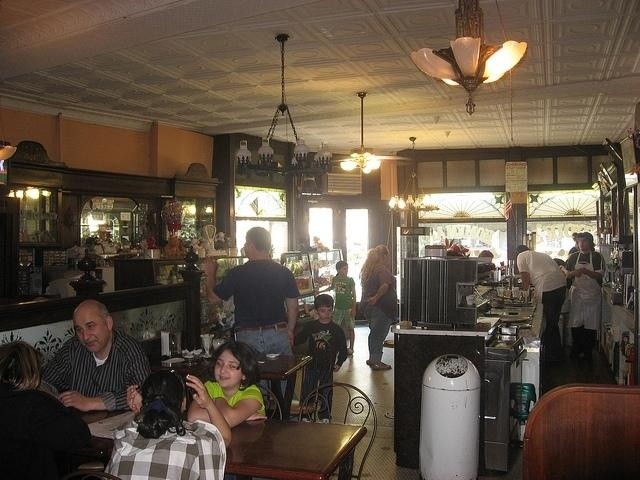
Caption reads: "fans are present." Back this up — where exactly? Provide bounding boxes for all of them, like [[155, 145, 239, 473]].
[[332, 155, 414, 170]]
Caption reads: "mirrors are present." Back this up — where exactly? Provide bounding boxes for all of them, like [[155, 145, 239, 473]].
[[80, 195, 142, 254]]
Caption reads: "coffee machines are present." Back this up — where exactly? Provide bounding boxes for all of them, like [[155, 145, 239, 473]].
[[443, 258, 495, 327]]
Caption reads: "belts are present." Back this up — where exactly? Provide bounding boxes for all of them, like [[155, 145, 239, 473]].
[[239, 324, 287, 331]]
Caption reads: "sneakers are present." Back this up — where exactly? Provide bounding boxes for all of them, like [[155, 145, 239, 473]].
[[303, 418, 312, 423], [372, 362, 392, 370], [315, 419, 329, 424], [347, 349, 355, 354]]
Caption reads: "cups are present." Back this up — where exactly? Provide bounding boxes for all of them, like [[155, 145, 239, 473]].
[[507, 268, 514, 276], [493, 270, 502, 283], [228, 248, 237, 258], [159, 329, 227, 368], [197, 248, 205, 258], [496, 286, 529, 304], [143, 249, 161, 260], [465, 295, 474, 306]]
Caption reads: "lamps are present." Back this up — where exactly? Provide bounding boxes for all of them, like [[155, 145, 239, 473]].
[[388, 138, 441, 211], [341, 91, 382, 174], [412, 0, 528, 115], [235, 35, 331, 176]]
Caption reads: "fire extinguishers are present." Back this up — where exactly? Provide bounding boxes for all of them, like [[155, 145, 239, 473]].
[[621, 341, 636, 386]]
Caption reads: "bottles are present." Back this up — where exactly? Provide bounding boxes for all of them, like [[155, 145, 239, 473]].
[[77, 249, 95, 272]]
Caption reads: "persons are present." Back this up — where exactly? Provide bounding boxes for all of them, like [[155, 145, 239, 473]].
[[187, 340, 267, 428], [1, 341, 92, 480], [105, 371, 232, 480], [205, 228, 299, 402], [477, 231, 606, 366], [316, 261, 356, 356], [361, 244, 397, 371], [35, 299, 149, 412], [295, 295, 348, 423]]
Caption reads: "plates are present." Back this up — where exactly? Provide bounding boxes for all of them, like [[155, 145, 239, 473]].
[[266, 354, 279, 359]]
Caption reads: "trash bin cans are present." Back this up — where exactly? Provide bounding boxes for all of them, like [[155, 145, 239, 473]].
[[419, 354, 480, 480]]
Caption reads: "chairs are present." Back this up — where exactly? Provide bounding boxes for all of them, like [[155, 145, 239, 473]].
[[257, 384, 283, 420], [63, 469, 121, 479], [297, 382, 378, 479], [291, 340, 333, 422]]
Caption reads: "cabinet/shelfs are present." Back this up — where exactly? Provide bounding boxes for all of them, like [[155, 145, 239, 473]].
[[114, 248, 343, 344]]
